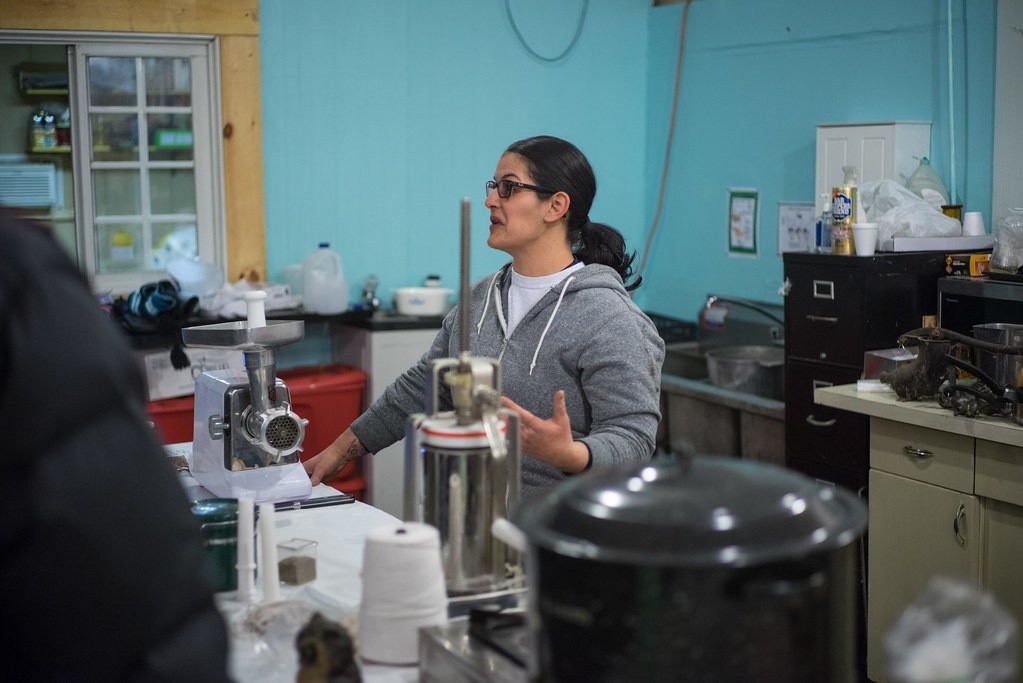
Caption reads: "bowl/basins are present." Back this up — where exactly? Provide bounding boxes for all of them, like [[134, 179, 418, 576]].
[[395, 286, 452, 315]]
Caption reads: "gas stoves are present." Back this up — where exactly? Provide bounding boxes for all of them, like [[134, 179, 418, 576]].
[[419, 608, 528, 683]]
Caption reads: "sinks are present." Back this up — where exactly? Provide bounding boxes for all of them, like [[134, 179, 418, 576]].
[[739, 377, 786, 467], [665, 365, 786, 461], [643, 349, 710, 444]]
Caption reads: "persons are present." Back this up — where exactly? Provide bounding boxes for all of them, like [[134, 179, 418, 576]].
[[303, 135, 666, 502], [0, 203, 235, 683]]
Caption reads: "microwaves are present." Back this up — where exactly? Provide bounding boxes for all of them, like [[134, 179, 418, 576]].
[[935, 276, 1022, 343]]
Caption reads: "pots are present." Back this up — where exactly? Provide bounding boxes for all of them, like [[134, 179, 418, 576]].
[[706, 344, 785, 398], [945, 352, 1023, 426]]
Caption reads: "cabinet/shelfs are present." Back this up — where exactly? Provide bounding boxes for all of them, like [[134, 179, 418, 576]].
[[815, 120, 932, 220], [867, 412, 1023, 683], [24, 89, 193, 153]]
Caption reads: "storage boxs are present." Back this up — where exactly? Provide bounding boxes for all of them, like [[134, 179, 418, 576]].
[[132, 340, 243, 402]]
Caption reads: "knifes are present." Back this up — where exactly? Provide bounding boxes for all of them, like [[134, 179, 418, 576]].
[[256, 493, 355, 512]]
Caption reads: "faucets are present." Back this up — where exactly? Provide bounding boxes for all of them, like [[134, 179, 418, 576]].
[[706, 296, 784, 347]]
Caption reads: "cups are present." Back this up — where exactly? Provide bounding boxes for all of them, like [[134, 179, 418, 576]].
[[964, 211, 986, 236], [852, 223, 878, 256], [193, 497, 260, 594], [989, 245, 1018, 273], [523, 449, 873, 683], [276, 537, 318, 584], [57, 123, 75, 147]]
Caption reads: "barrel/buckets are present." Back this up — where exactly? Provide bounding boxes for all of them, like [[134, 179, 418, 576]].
[[322, 476, 365, 502], [973, 323, 1023, 378], [275, 363, 367, 480], [147, 397, 195, 444]]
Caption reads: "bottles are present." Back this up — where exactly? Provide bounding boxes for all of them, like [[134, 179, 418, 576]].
[[839, 164, 866, 223], [92, 115, 112, 151], [305, 242, 349, 314], [30, 113, 56, 147], [108, 227, 133, 262], [904, 156, 952, 207], [358, 275, 379, 313]]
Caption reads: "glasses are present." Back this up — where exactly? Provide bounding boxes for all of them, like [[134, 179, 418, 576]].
[[486, 180, 567, 219]]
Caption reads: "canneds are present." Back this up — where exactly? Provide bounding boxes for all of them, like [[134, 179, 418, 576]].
[[830, 187, 857, 254]]
